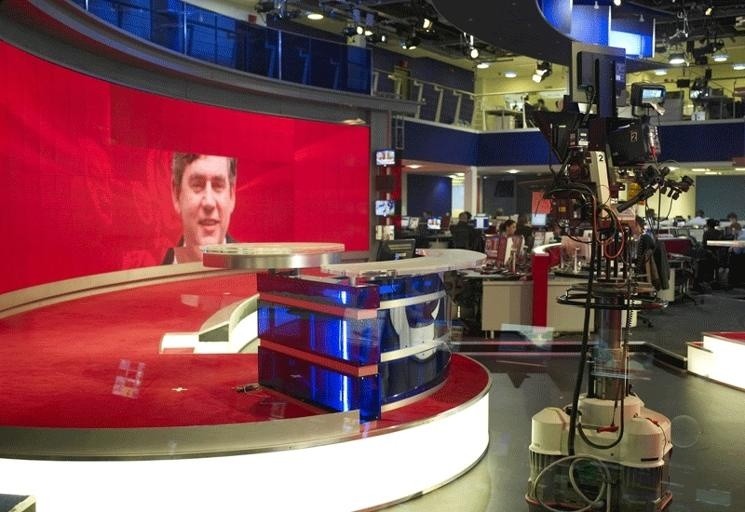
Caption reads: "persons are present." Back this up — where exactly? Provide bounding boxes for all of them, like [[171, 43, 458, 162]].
[[703, 219, 721, 252], [496, 220, 516, 268], [534, 98, 548, 112], [686, 209, 706, 227], [516, 213, 534, 252], [524, 95, 533, 115], [636, 215, 645, 236], [159, 151, 240, 269], [449, 213, 481, 249], [727, 212, 737, 224], [728, 222, 745, 254]]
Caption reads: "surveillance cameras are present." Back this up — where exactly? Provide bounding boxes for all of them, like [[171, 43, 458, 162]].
[[485, 181, 488, 184]]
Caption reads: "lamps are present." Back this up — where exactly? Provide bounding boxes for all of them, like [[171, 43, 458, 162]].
[[401, 28, 421, 51], [289, 8, 323, 23], [346, 9, 387, 44], [463, 33, 479, 62], [667, 37, 688, 66], [710, 28, 730, 64], [530, 58, 554, 82]]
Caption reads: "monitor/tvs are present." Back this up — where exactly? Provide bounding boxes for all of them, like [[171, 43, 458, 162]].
[[474, 217, 489, 229], [407, 173, 453, 218], [426, 217, 441, 229], [375, 176, 395, 192], [494, 180, 513, 197], [530, 191, 555, 213], [375, 225, 396, 240], [374, 149, 399, 167], [608, 4, 655, 58], [409, 217, 419, 228], [571, 44, 626, 108], [401, 216, 409, 228], [531, 213, 547, 225], [374, 200, 397, 217], [376, 239, 416, 262], [694, 175, 745, 221], [630, 83, 666, 107]]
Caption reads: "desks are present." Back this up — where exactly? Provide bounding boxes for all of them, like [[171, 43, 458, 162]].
[[707, 239, 745, 283], [481, 275, 638, 339], [690, 95, 736, 120]]
